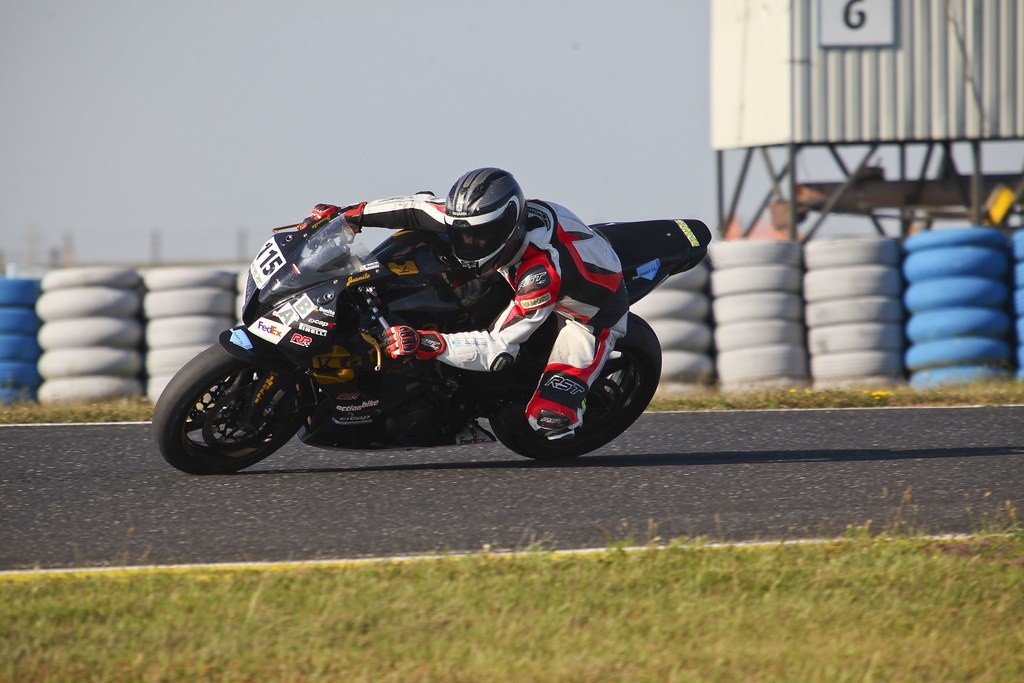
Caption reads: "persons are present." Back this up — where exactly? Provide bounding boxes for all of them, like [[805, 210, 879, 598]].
[[301, 169, 628, 441]]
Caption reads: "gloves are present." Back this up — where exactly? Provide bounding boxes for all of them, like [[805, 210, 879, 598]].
[[307, 201, 367, 235], [380, 326, 448, 363]]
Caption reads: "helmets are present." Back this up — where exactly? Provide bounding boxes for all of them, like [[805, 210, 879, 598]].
[[443, 167, 527, 278]]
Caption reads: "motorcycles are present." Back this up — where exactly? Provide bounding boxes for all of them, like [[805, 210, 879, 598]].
[[150, 201, 713, 476]]
